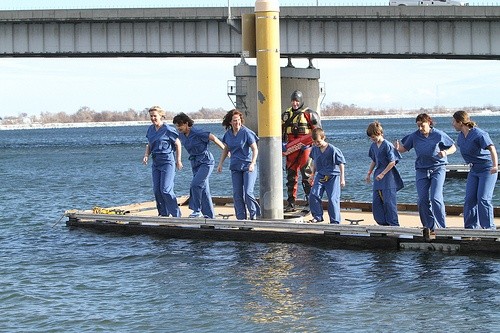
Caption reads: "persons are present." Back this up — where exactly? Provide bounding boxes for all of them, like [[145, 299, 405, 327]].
[[394, 112, 457, 232], [308, 128, 346, 224], [281, 90, 323, 217], [172, 112, 231, 219], [141, 106, 183, 218], [216, 109, 262, 220], [366, 121, 404, 226], [452, 111, 498, 229]]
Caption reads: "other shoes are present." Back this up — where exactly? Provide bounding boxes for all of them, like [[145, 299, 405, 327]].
[[309, 217, 323, 223], [189, 212, 200, 218], [285, 203, 294, 211], [303, 205, 310, 212]]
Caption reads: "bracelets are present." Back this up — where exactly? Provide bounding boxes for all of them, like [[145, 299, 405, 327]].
[[381, 172, 385, 177], [444, 150, 447, 156], [310, 172, 315, 177]]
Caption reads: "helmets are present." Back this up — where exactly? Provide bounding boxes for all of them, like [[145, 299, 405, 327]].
[[291, 90, 304, 109]]
